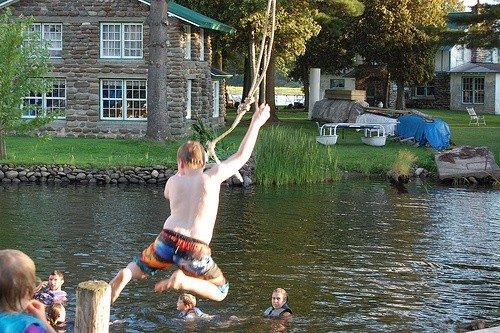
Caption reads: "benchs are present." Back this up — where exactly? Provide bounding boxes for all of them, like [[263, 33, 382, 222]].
[[316, 123, 387, 147]]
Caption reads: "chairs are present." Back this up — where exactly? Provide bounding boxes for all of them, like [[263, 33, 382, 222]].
[[466, 106, 486, 126]]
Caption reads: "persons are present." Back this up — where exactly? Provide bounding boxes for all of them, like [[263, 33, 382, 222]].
[[109, 102, 292, 320], [0, 249, 69, 333]]
